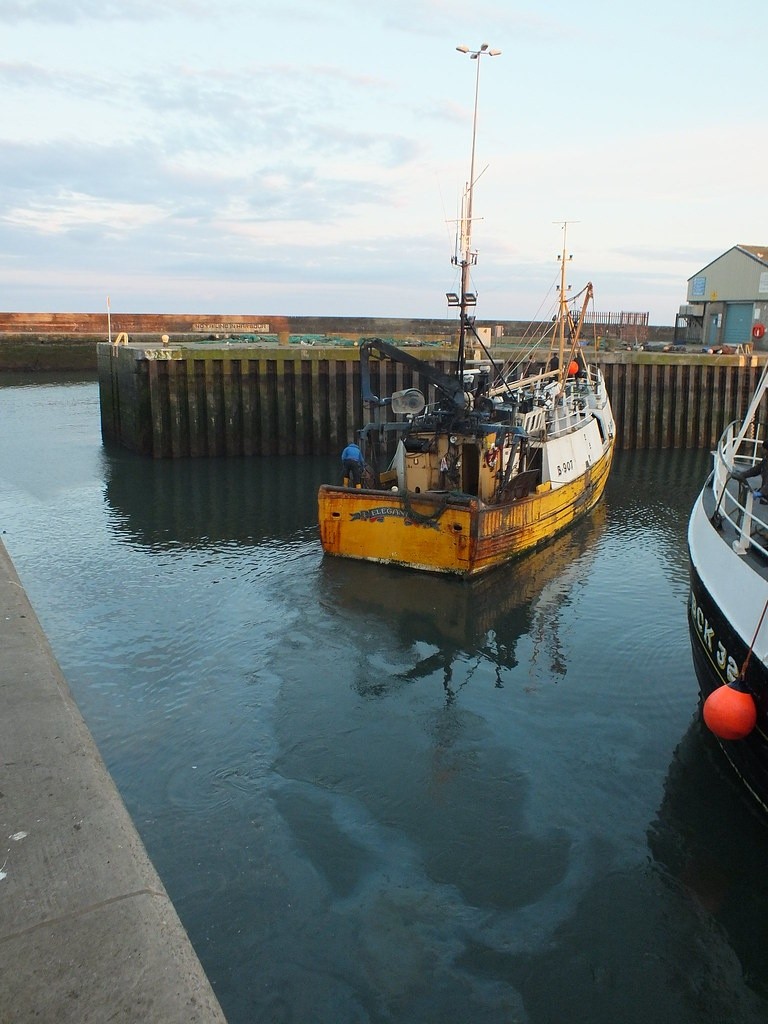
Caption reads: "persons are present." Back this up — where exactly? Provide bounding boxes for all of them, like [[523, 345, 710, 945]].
[[574, 356, 584, 379], [341, 443, 362, 488], [548, 353, 558, 382]]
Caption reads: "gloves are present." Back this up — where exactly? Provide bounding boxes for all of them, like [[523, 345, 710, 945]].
[[753, 491, 762, 500]]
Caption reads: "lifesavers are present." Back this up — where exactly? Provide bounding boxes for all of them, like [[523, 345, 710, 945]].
[[488, 443, 499, 468], [753, 324, 764, 339]]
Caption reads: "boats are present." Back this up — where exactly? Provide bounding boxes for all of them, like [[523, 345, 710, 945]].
[[319, 160, 617, 579], [313, 488, 604, 710], [686, 352, 768, 841]]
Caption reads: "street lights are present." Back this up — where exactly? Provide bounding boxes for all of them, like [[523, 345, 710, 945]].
[[456, 42, 502, 346]]
